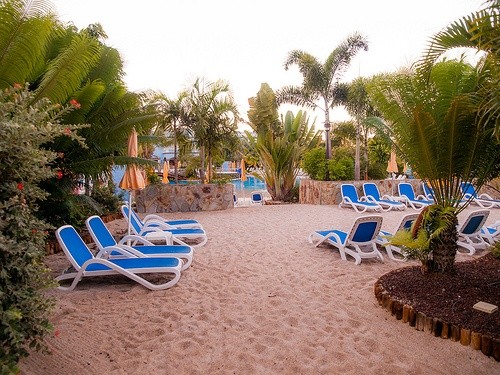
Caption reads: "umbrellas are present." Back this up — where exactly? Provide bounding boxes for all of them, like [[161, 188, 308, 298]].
[[240, 159, 247, 182], [162, 162, 169, 184], [119, 126, 145, 247], [386, 146, 399, 196]]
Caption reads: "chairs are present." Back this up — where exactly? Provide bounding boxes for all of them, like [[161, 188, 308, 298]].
[[51, 201, 208, 298], [233, 192, 264, 209], [308, 181, 500, 267]]
